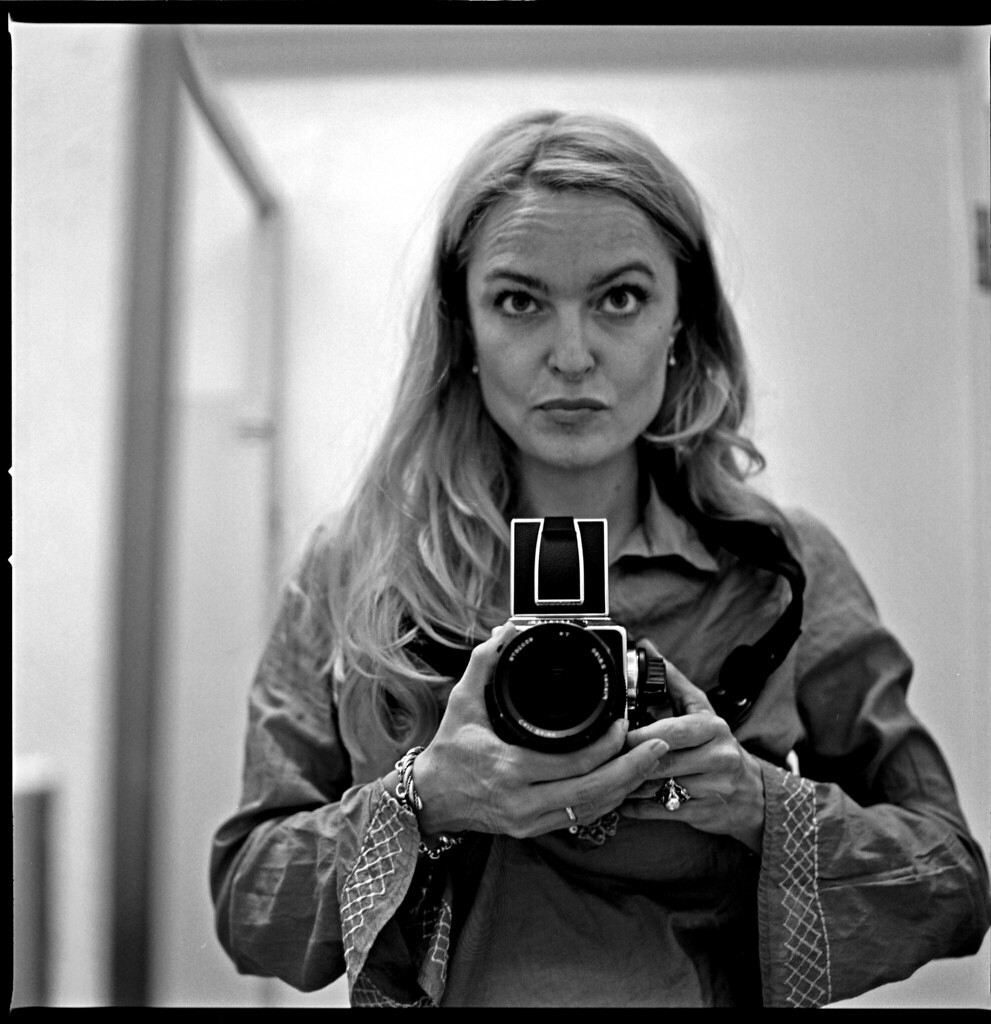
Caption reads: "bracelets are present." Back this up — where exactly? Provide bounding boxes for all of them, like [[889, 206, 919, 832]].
[[394, 745, 464, 860]]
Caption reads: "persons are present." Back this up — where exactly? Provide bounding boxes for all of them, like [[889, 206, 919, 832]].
[[211, 108, 991, 1008]]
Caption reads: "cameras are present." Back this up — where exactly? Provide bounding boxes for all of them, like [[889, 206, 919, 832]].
[[480, 513, 670, 759]]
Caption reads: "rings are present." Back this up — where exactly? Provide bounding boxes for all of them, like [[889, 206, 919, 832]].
[[656, 777, 692, 811], [565, 805, 579, 833]]
